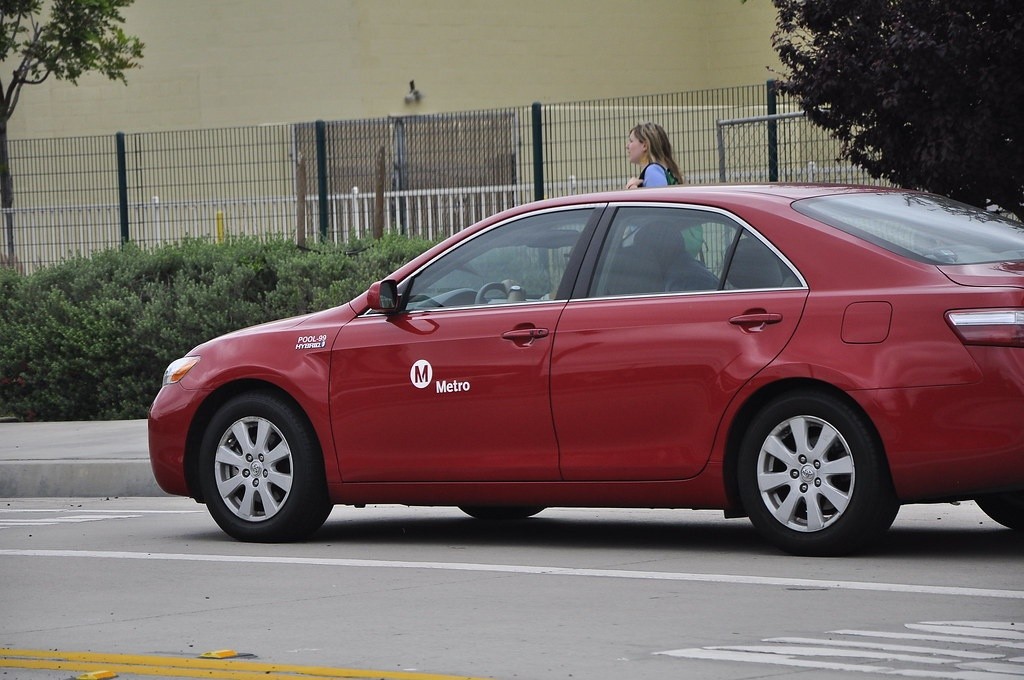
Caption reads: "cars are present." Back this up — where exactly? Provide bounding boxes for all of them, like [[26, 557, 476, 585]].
[[144, 181, 1024, 559]]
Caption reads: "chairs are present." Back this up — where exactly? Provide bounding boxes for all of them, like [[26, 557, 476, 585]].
[[609, 242, 664, 296], [725, 240, 785, 289]]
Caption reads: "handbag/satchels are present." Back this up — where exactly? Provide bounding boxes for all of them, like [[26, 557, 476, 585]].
[[637, 163, 679, 186]]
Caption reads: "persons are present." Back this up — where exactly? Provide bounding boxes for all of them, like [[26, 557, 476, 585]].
[[624, 123, 683, 189], [633, 220, 721, 292], [502, 279, 558, 303]]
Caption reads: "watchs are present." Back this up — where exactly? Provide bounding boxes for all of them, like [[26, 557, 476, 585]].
[[507, 285, 523, 296]]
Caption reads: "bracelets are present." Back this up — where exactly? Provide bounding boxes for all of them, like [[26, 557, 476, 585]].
[[628, 184, 634, 189]]
